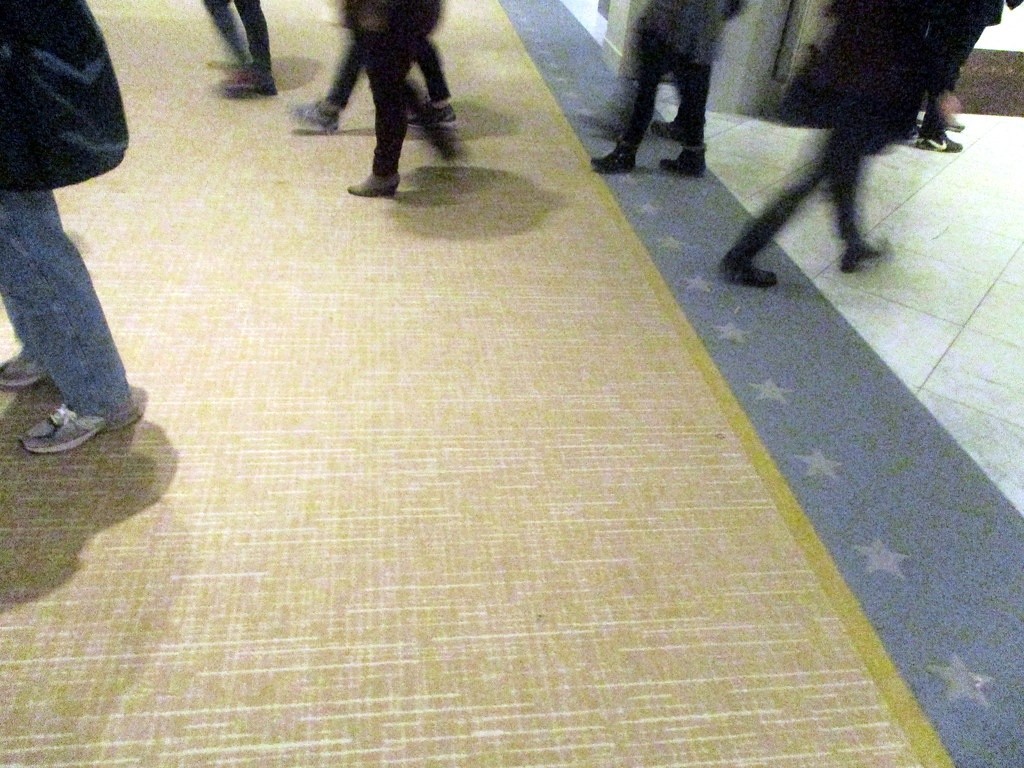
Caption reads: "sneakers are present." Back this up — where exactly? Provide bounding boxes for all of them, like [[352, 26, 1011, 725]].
[[18, 397, 141, 455], [916, 133, 963, 153], [0, 348, 48, 388]]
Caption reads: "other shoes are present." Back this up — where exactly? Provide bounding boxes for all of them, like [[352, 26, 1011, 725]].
[[944, 118, 965, 133], [221, 68, 278, 99], [287, 99, 339, 132], [407, 101, 458, 129], [650, 119, 682, 140], [841, 238, 892, 274], [718, 259, 777, 289]]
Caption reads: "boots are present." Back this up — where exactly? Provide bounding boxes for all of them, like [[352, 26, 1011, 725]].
[[348, 172, 399, 199], [590, 134, 640, 173], [659, 145, 707, 177]]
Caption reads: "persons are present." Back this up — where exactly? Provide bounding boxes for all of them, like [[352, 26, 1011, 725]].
[[205, 0, 277, 98], [576, 0, 745, 175], [717, 0, 961, 288], [894, 0, 1024, 153], [0, 0, 135, 453], [290, 0, 459, 196]]
